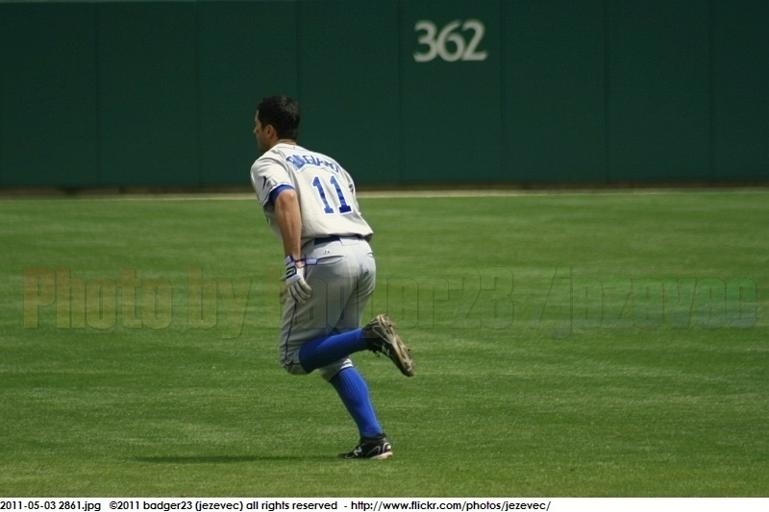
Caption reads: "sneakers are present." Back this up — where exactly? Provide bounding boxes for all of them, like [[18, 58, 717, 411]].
[[363, 314, 416, 377], [344, 432, 393, 460]]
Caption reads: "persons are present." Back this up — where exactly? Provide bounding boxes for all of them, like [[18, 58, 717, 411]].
[[249, 95, 417, 458]]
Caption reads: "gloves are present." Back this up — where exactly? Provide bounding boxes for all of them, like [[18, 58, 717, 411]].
[[283, 255, 314, 306]]
